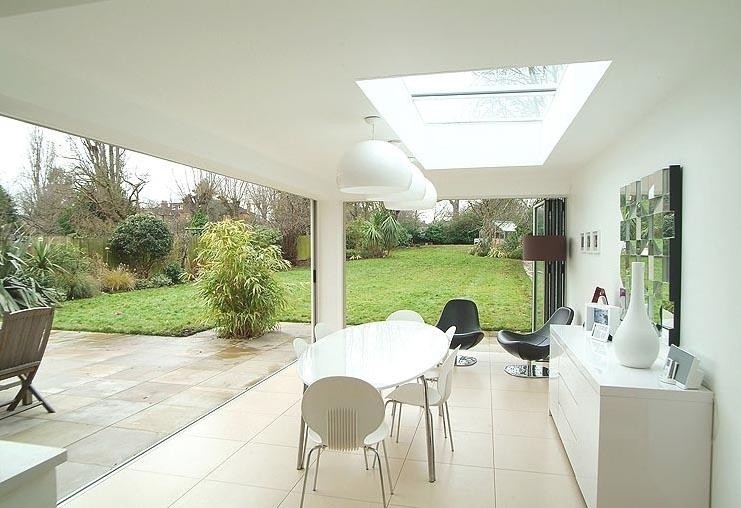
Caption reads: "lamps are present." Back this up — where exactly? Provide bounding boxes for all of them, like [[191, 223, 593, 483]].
[[338, 115, 438, 210], [522, 233, 569, 261]]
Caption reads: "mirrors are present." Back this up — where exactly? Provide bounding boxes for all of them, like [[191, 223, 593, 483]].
[[618, 164, 684, 362]]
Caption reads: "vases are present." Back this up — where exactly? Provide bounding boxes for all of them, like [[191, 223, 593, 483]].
[[613, 260, 660, 369]]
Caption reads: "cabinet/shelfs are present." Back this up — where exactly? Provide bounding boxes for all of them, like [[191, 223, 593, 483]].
[[549, 323, 714, 508]]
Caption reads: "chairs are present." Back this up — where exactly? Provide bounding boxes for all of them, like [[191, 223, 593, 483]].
[[1, 302, 56, 420], [497, 306, 575, 379]]
